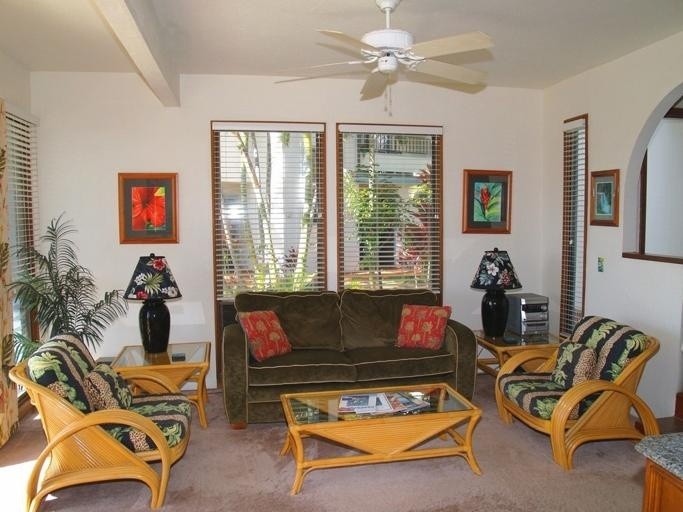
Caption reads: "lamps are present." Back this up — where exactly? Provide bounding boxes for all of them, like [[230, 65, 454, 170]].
[[471, 248, 523, 338], [122, 254, 182, 352], [378, 55, 396, 73]]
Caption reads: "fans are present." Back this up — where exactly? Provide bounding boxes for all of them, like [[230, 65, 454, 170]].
[[275, 0, 496, 84]]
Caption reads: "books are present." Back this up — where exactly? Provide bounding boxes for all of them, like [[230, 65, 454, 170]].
[[336, 391, 430, 415]]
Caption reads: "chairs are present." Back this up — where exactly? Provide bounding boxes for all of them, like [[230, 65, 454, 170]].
[[494, 315, 661, 470], [7, 333, 193, 512]]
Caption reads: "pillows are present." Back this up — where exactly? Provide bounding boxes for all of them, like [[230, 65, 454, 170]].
[[236, 310, 291, 363], [552, 341, 598, 391], [84, 363, 132, 412], [395, 303, 452, 350]]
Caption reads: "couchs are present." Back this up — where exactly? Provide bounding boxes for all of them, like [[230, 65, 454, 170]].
[[222, 287, 478, 430]]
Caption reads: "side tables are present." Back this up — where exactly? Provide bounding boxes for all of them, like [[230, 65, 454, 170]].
[[111, 341, 213, 429], [471, 330, 566, 377]]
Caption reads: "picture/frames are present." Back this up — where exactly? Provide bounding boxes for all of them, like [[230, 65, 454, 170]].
[[590, 169, 619, 227], [461, 168, 512, 235], [117, 173, 179, 244]]
